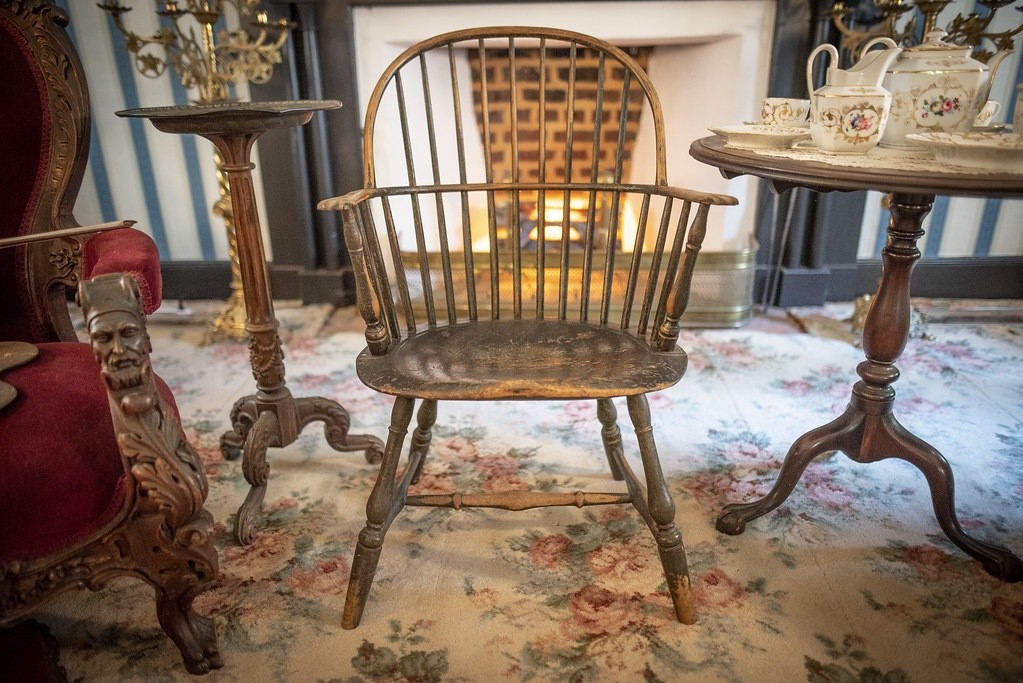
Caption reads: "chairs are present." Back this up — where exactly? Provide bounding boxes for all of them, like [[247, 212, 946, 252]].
[[1, 2, 228, 675], [310, 25, 739, 628]]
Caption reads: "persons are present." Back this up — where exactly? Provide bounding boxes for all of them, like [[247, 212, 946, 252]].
[[87, 310, 149, 390]]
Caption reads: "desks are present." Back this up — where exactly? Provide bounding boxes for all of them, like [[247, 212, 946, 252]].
[[685, 125, 1022, 586], [109, 99, 386, 545]]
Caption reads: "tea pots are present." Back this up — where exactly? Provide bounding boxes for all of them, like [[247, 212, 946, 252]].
[[861, 26, 1016, 151]]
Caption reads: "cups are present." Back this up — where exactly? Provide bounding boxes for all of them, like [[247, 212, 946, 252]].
[[973, 101, 1001, 126], [761, 97, 810, 126]]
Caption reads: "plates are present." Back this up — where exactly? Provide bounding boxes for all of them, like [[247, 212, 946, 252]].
[[708, 125, 811, 150], [906, 134, 1023, 169]]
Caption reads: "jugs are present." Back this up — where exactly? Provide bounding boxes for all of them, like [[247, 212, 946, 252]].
[[807, 43, 904, 155]]
